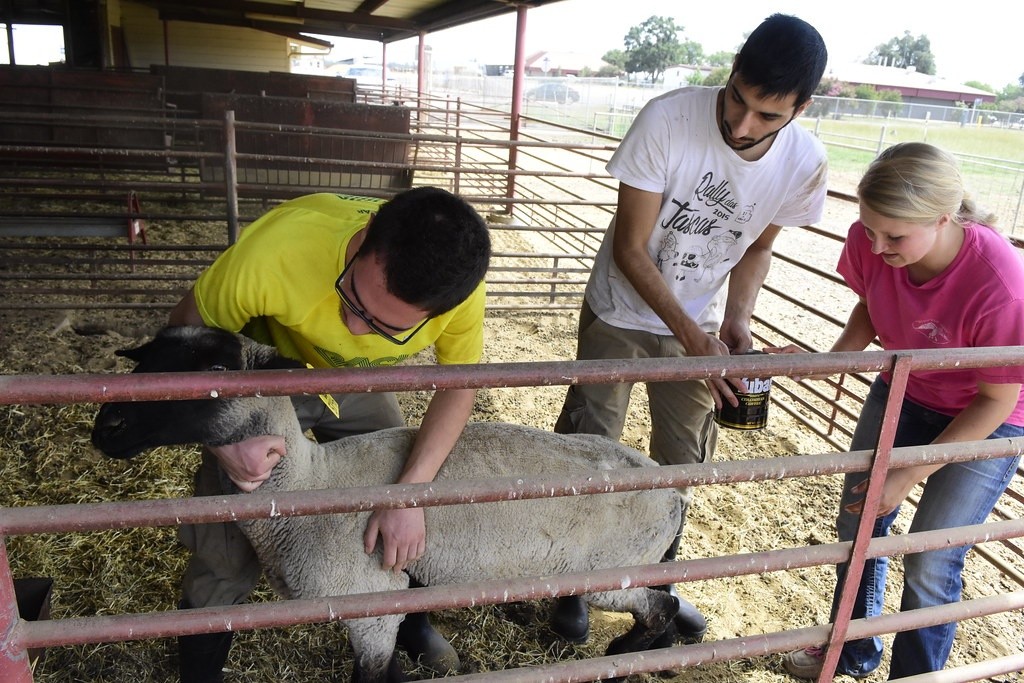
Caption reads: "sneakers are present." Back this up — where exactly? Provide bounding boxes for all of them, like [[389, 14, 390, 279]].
[[783, 643, 846, 678]]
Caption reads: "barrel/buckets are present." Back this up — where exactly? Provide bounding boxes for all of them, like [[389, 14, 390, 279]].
[[712, 349, 772, 429]]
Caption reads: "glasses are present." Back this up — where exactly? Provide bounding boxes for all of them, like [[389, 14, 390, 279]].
[[334, 249, 436, 345]]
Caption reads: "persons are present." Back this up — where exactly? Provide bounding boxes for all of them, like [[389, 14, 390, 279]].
[[760, 141, 1024, 680], [549, 12, 829, 644], [167, 185, 492, 683]]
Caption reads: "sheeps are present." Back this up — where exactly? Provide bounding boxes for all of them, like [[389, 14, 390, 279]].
[[91, 322, 693, 683]]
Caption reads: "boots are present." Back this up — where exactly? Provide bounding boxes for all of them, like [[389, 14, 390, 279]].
[[397, 569, 462, 677], [177, 599, 234, 683], [646, 502, 708, 635], [554, 595, 591, 643]]
